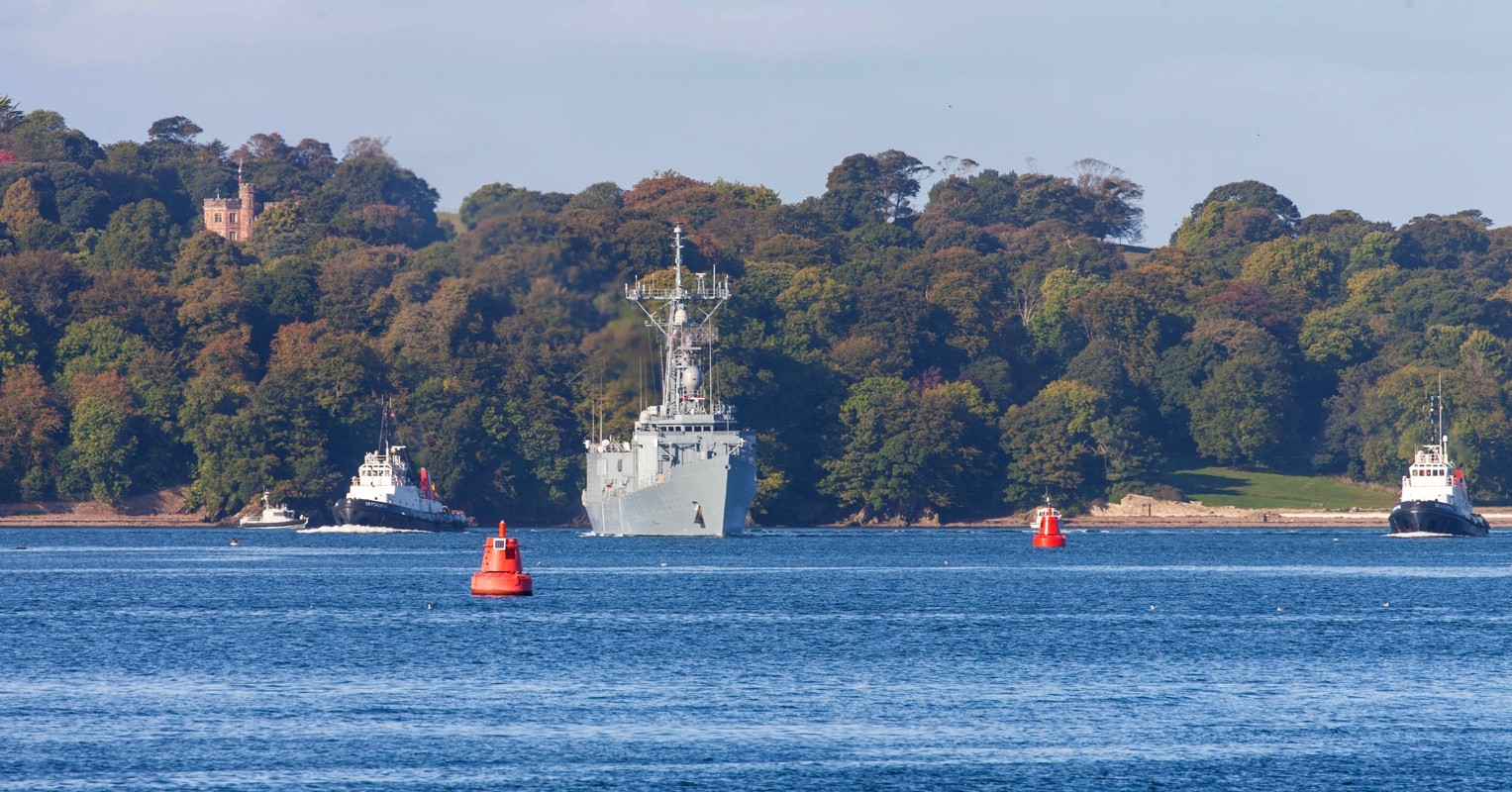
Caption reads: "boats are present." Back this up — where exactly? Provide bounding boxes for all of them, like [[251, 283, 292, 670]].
[[1388, 372, 1492, 537], [1029, 486, 1064, 529], [331, 395, 466, 534], [582, 224, 758, 539], [239, 484, 309, 529]]
